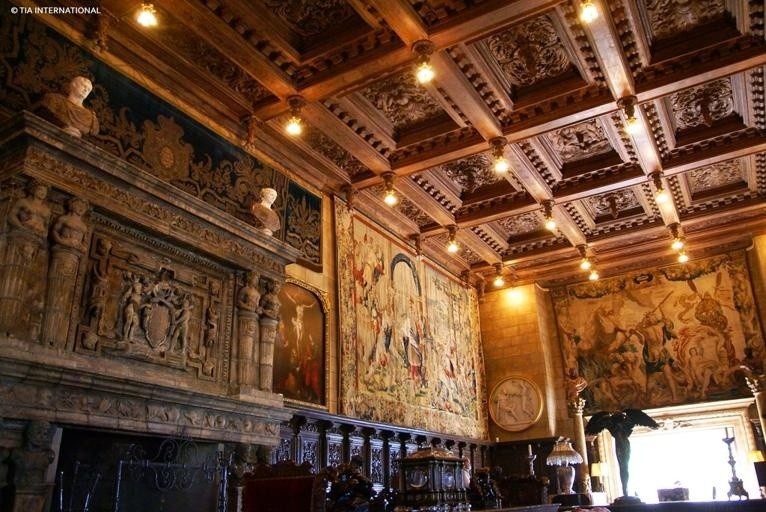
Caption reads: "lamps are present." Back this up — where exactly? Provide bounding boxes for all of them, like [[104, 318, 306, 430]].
[[129, 1, 691, 289], [546, 440, 584, 494]]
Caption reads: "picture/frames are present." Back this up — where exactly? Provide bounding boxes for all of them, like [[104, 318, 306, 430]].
[[273, 274, 332, 414]]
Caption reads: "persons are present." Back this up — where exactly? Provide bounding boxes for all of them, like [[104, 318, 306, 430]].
[[275, 290, 322, 405], [40, 75, 100, 141], [516, 381, 534, 420], [250, 186, 281, 236], [122, 282, 152, 343], [205, 279, 223, 348], [1, 179, 52, 239], [42, 194, 91, 254], [233, 443, 273, 485], [10, 421, 56, 489], [83, 236, 113, 350], [165, 298, 191, 357], [496, 387, 518, 420], [597, 410, 634, 498], [566, 269, 762, 404], [258, 279, 283, 319], [237, 269, 261, 312]]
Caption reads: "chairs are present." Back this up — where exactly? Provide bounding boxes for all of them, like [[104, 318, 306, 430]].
[[237, 458, 332, 512]]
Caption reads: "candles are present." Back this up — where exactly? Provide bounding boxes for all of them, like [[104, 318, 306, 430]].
[[724, 427, 728, 438], [528, 444, 533, 455]]
